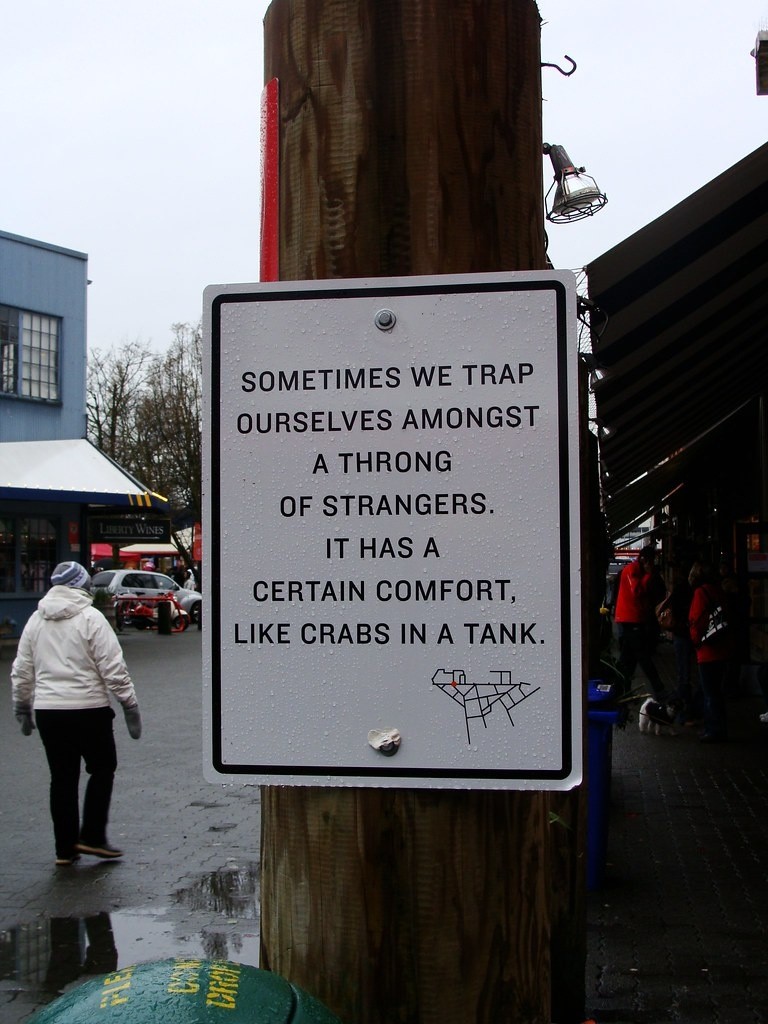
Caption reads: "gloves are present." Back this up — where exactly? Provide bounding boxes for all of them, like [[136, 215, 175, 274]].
[[124, 706, 143, 739], [13, 702, 36, 737]]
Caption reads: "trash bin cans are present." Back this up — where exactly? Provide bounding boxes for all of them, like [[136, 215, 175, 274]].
[[587, 679, 619, 873]]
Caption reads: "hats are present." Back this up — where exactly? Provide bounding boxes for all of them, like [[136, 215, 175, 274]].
[[51, 561, 92, 590]]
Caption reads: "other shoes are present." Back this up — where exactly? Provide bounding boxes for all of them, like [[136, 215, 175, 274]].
[[697, 727, 728, 745], [75, 838, 124, 859], [760, 712, 768, 722], [56, 852, 81, 866]]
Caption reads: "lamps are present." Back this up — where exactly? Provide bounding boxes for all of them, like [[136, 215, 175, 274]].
[[576, 294, 618, 446], [542, 142, 609, 226]]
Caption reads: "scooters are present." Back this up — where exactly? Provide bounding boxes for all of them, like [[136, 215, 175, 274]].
[[109, 590, 191, 634]]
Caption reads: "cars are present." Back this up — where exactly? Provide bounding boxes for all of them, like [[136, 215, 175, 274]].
[[90, 569, 203, 631]]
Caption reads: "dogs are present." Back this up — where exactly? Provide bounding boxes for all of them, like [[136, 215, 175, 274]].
[[638, 697, 679, 737]]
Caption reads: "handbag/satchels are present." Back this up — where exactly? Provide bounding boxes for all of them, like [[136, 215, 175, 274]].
[[700, 587, 729, 644]]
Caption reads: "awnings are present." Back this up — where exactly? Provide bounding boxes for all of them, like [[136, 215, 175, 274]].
[[581, 139, 768, 563], [90, 544, 181, 560], [0, 437, 170, 514]]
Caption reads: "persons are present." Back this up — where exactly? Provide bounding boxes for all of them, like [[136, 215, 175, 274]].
[[11, 559, 144, 865], [142, 560, 196, 592], [596, 542, 754, 710], [686, 561, 736, 746]]
